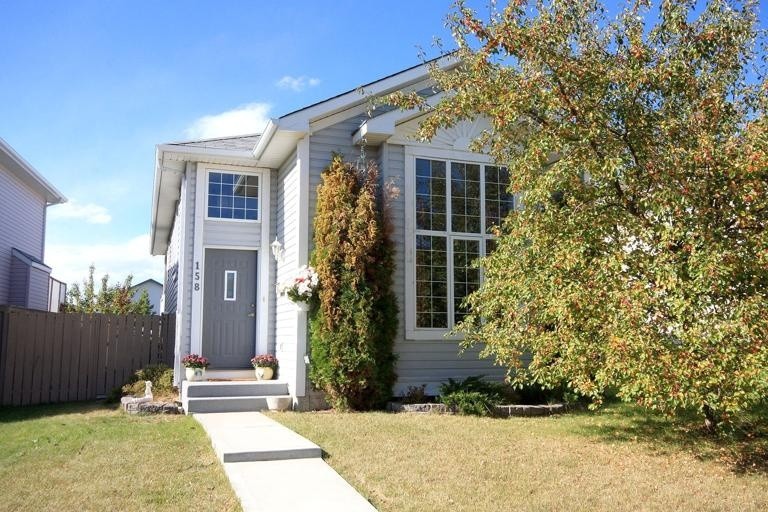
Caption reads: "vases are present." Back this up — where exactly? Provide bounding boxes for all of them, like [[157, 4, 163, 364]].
[[185, 366, 206, 382], [292, 298, 313, 312], [254, 366, 273, 380]]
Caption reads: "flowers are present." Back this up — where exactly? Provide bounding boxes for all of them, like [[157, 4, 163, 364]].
[[251, 353, 278, 369], [285, 268, 318, 303], [182, 353, 210, 369]]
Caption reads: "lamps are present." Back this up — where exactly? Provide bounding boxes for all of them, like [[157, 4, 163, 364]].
[[270, 232, 285, 260]]
